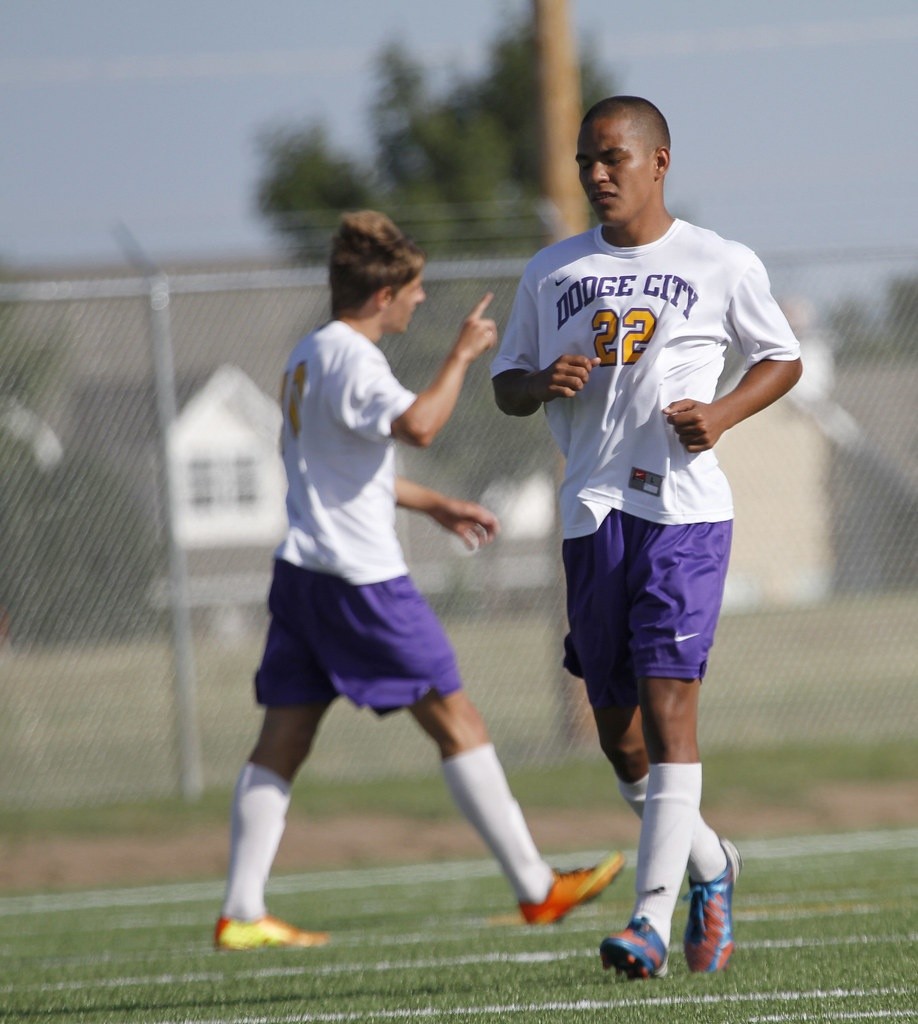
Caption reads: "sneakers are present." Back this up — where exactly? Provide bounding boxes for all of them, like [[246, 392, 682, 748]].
[[601, 910, 668, 978], [518, 852, 624, 925], [214, 907, 328, 950], [681, 837, 741, 973]]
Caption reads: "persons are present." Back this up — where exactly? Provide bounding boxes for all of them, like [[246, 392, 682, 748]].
[[492, 96, 803, 980], [215, 209, 625, 952]]
